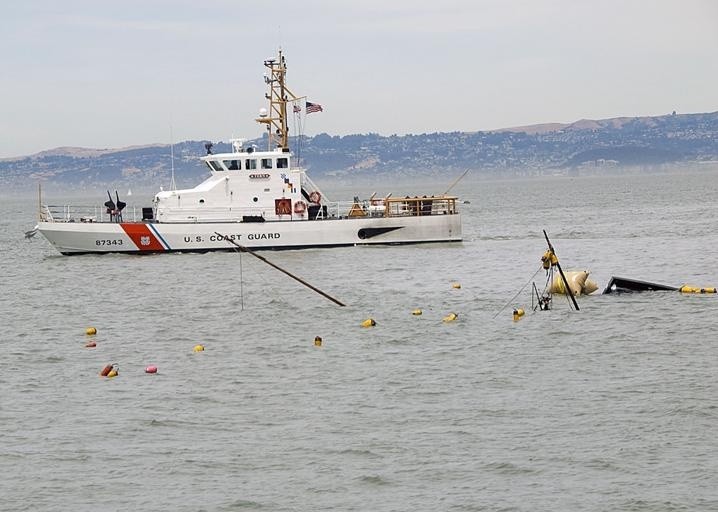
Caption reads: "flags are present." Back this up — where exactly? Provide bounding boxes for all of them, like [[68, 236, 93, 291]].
[[294, 106, 298, 112], [297, 106, 301, 111], [306, 102, 322, 113]]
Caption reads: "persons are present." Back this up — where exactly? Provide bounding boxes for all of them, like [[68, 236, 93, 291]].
[[229, 160, 237, 169], [405, 196, 431, 215]]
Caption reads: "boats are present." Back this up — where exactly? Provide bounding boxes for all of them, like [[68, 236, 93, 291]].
[[26, 48, 470, 258]]
